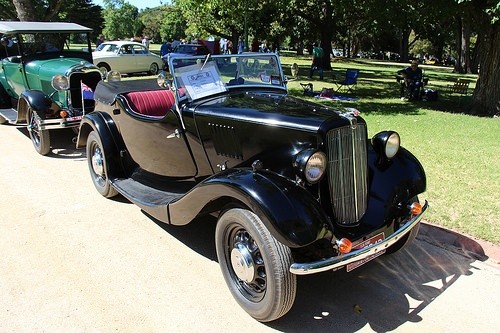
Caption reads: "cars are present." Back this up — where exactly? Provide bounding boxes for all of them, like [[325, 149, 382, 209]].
[[163, 44, 223, 70], [0, 20, 104, 156], [74, 50, 429, 323], [91, 40, 164, 82]]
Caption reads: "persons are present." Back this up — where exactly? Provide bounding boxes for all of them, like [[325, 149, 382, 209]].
[[400, 61, 423, 103], [308, 42, 325, 81], [97, 33, 275, 57]]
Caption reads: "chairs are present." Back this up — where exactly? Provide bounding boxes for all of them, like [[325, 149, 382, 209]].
[[401, 74, 425, 99], [445, 79, 470, 100], [300, 83, 312, 95], [335, 68, 360, 96]]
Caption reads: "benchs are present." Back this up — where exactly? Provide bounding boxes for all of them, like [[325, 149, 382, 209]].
[[126, 88, 184, 118]]
